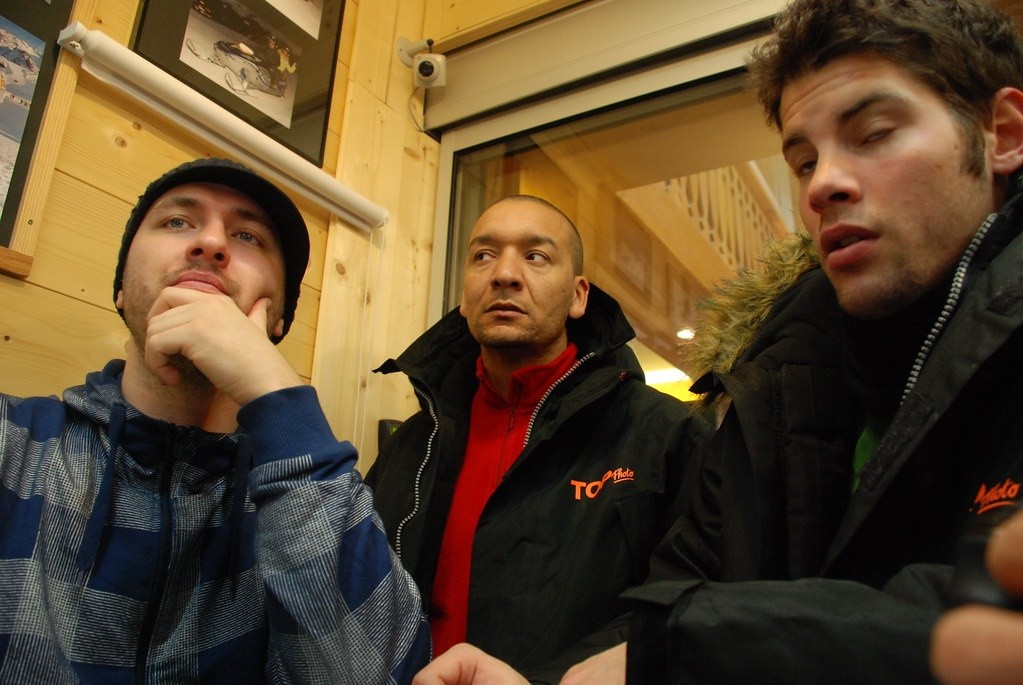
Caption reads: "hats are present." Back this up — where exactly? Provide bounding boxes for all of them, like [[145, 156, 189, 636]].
[[113, 157, 310, 345]]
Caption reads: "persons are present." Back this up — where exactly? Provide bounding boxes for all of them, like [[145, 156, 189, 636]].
[[388, 1, 1023, 685], [361, 193, 697, 685], [233, 33, 297, 91], [1, 156, 433, 685]]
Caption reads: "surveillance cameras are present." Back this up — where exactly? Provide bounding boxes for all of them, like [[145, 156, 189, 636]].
[[412, 52, 446, 88]]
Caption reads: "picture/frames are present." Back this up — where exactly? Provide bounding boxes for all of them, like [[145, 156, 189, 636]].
[[132, 0, 347, 164]]
